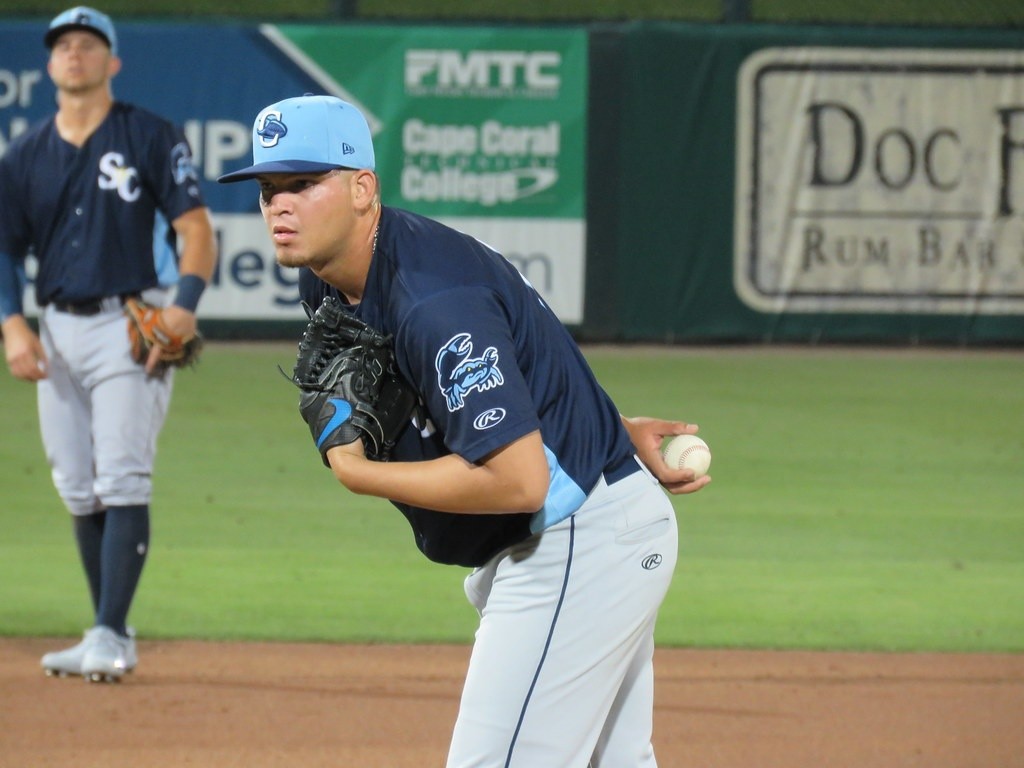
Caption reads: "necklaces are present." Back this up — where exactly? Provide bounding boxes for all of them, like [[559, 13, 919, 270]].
[[372, 225, 379, 254]]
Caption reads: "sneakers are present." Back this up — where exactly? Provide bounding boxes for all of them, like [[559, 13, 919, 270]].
[[41, 626, 137, 684]]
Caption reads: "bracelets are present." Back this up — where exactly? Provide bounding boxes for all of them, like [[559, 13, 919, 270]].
[[173, 274, 206, 314]]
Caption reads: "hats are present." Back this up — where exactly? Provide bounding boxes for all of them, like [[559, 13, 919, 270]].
[[43, 5, 117, 57], [216, 95, 376, 184]]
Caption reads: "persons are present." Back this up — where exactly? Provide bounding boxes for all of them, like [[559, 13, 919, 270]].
[[0, 6, 218, 685], [216, 93, 712, 768]]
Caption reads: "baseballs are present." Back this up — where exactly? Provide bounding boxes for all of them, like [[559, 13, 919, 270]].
[[663, 433, 712, 481]]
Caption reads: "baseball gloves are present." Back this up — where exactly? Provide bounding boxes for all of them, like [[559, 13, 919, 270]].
[[121, 296, 204, 380], [276, 297, 397, 468]]
